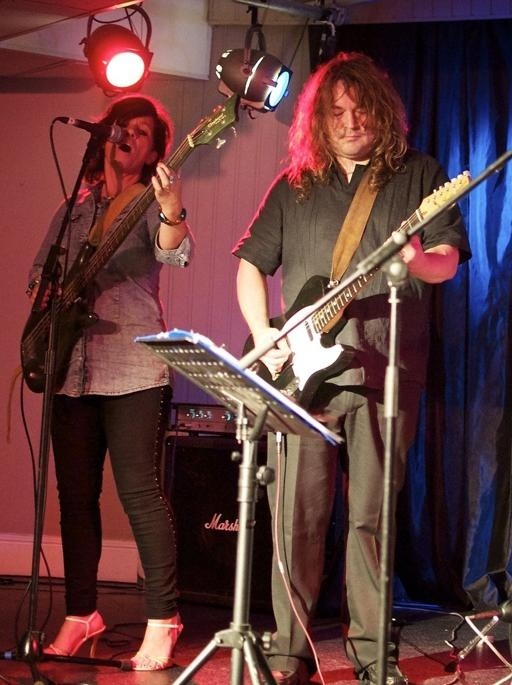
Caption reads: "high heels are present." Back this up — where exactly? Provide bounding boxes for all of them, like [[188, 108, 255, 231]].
[[44, 610, 107, 658], [130, 623, 184, 670]]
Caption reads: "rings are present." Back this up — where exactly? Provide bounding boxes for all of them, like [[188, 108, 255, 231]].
[[169, 176, 177, 185]]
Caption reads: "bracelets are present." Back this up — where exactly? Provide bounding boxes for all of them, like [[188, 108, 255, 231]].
[[159, 209, 186, 227]]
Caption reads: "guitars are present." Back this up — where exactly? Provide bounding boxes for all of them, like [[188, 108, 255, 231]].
[[20, 93, 239, 393], [243, 171, 471, 410]]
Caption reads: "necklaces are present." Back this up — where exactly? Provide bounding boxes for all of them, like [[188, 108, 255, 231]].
[[96, 189, 117, 203]]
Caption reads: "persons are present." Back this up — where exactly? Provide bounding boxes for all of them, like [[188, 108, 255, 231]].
[[29, 94, 196, 670], [232, 52, 472, 685]]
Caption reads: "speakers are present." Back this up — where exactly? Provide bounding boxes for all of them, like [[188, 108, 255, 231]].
[[161, 435, 342, 619]]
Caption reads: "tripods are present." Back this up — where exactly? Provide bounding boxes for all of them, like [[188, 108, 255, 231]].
[[170, 430, 279, 685], [0, 134, 132, 685]]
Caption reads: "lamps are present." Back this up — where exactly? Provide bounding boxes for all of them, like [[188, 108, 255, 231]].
[[215, 6, 293, 117], [78, 4, 153, 97]]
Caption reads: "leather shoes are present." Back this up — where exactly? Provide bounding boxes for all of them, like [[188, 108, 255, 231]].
[[260, 669, 298, 684], [364, 658, 409, 685]]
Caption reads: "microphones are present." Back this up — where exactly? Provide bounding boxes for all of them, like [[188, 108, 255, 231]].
[[387, 259, 408, 284], [54, 116, 124, 144]]
